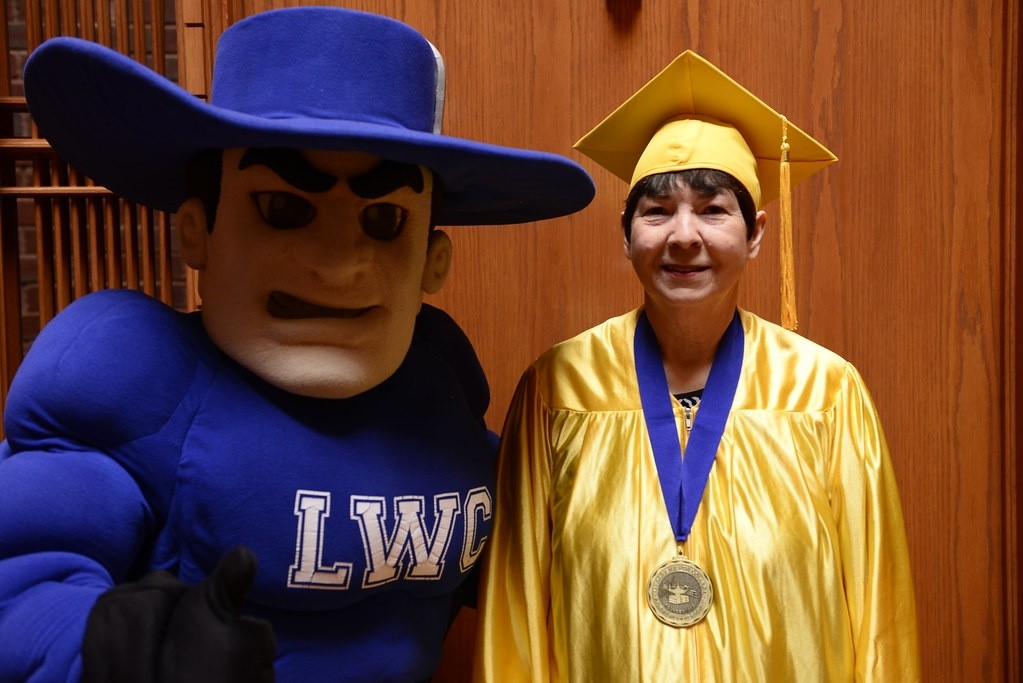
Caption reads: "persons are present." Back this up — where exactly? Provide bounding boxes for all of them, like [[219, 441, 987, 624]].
[[472, 49, 921, 683], [0, 5, 597, 683]]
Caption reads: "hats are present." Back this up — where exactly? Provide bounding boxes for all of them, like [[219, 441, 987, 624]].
[[570, 48, 838, 330]]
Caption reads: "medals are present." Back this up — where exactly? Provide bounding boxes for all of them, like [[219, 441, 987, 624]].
[[646, 558, 714, 628]]
